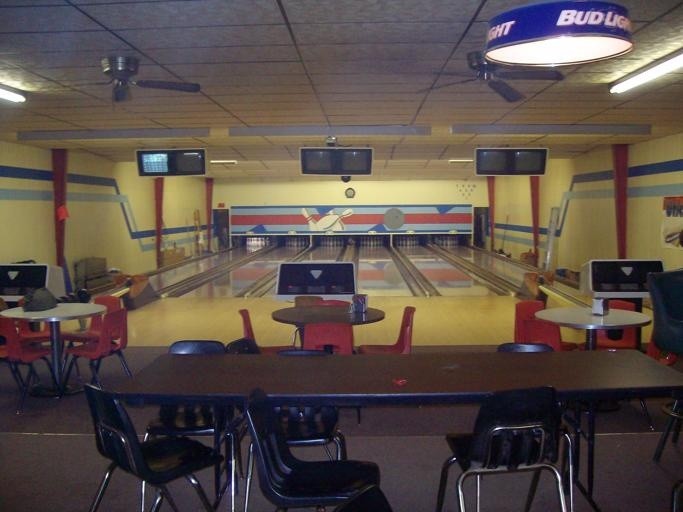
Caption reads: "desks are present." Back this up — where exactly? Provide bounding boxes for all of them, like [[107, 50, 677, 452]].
[[112, 348, 682, 511]]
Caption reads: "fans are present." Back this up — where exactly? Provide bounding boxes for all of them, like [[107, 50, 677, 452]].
[[412, 49, 566, 103], [61, 55, 200, 104]]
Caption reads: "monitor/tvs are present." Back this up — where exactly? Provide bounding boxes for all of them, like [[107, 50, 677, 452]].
[[512, 148, 549, 177], [337, 145, 375, 177], [171, 149, 207, 176], [137, 149, 172, 177], [299, 147, 337, 176], [473, 147, 513, 176]]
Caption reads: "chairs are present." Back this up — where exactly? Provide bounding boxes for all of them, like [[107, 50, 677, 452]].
[[0, 294, 132, 416], [236, 293, 421, 423], [511, 269, 683, 460]]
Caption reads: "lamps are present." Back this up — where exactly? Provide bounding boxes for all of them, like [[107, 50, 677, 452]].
[[482, 1, 635, 70]]
